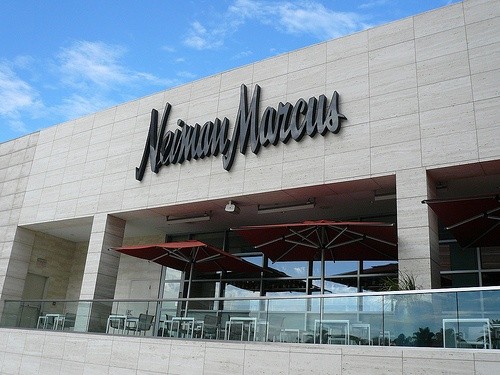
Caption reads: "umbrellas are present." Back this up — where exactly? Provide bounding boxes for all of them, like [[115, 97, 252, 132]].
[[423, 193, 500, 247], [323, 263, 398, 338], [229, 220, 397, 344], [228, 266, 333, 341], [110, 240, 271, 339]]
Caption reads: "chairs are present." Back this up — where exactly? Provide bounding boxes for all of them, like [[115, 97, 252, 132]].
[[107, 313, 154, 336], [224, 317, 286, 342], [161, 314, 222, 339], [300, 318, 372, 344], [36, 311, 76, 331]]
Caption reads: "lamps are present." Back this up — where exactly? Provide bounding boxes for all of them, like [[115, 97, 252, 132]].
[[257, 197, 316, 216], [166, 211, 213, 225], [373, 182, 449, 201]]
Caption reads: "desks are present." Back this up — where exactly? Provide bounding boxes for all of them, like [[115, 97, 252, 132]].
[[106, 316, 139, 335], [442, 318, 491, 348], [43, 313, 64, 330], [169, 317, 204, 339], [227, 317, 264, 341], [279, 328, 299, 343], [313, 319, 352, 344]]
